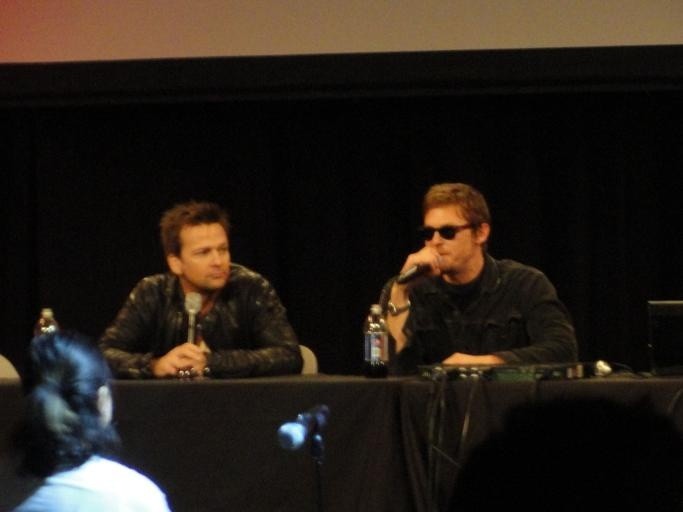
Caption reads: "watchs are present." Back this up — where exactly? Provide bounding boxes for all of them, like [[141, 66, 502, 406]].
[[387, 301, 410, 316]]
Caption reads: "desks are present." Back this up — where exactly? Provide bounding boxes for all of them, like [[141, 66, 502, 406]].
[[1, 374, 683, 512]]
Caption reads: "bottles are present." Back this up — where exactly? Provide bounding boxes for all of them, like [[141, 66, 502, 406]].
[[33, 306, 60, 341], [363, 304, 390, 379]]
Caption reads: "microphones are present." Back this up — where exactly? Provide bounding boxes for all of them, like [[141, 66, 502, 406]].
[[397, 265, 431, 285], [183, 292, 201, 344], [277, 405, 329, 451]]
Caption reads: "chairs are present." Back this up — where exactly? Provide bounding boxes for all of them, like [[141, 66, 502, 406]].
[[299, 343, 320, 375]]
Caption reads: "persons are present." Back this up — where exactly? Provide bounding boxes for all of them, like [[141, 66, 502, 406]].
[[362, 182, 579, 376], [1, 329, 172, 512], [96, 200, 303, 380]]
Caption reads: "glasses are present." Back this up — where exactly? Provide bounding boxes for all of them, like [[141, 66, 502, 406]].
[[420, 225, 473, 240]]
